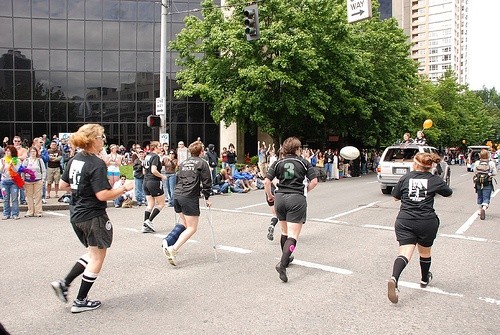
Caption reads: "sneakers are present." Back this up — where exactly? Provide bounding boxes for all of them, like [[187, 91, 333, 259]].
[[285, 255, 295, 267], [50, 278, 71, 303], [275, 262, 288, 282], [387, 276, 400, 303], [420, 272, 433, 288], [141, 226, 153, 233], [144, 218, 156, 232], [164, 245, 179, 266], [70, 296, 102, 312], [161, 238, 168, 256]]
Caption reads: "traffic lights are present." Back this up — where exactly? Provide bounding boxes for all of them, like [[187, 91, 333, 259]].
[[244, 4, 260, 42], [147, 114, 159, 127]]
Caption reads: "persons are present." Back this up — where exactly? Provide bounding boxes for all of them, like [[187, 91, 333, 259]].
[[198, 137, 347, 197], [0, 134, 191, 219], [393, 131, 426, 159], [161, 141, 212, 265], [343, 148, 383, 178], [50, 123, 133, 313], [473, 149, 498, 220], [141, 141, 167, 234], [265, 138, 318, 282], [443, 139, 500, 169], [388, 153, 453, 303]]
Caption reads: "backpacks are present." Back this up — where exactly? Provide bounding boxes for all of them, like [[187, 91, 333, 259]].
[[473, 161, 493, 189]]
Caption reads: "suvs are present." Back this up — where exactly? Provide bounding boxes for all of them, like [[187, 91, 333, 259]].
[[467, 145, 492, 171], [377, 144, 452, 195]]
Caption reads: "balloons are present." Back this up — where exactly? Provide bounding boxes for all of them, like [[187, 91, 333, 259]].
[[423, 119, 432, 129]]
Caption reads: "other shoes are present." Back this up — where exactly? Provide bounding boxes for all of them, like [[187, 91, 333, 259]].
[[480, 209, 486, 220], [266, 225, 274, 241]]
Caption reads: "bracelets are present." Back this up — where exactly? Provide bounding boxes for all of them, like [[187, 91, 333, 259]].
[[123, 185, 127, 193]]
